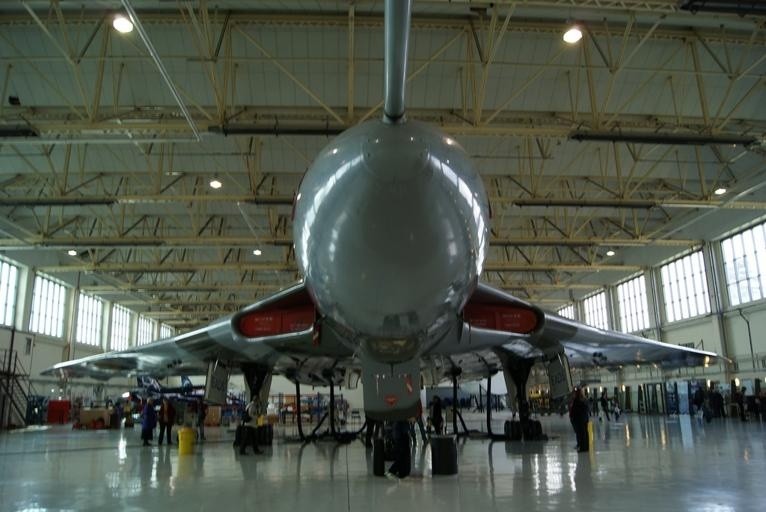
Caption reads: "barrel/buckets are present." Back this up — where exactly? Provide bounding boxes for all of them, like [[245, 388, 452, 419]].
[[178, 428, 195, 455], [431, 434, 458, 477]]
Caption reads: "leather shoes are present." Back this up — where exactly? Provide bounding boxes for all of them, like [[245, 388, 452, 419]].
[[255, 450, 264, 455], [240, 452, 249, 456]]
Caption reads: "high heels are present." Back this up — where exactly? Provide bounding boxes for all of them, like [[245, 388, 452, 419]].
[[143, 441, 152, 447]]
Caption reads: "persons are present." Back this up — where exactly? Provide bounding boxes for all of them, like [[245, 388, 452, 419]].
[[567, 384, 622, 452], [141, 396, 157, 447], [159, 397, 176, 445], [108, 399, 133, 420], [692, 384, 766, 422], [385, 400, 422, 483], [193, 396, 208, 440], [432, 395, 442, 435], [239, 395, 263, 455]]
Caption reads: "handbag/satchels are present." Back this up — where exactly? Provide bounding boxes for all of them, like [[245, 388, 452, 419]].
[[242, 411, 252, 422]]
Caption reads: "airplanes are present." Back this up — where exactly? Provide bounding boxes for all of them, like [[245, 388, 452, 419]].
[[38, 0, 735, 478]]
